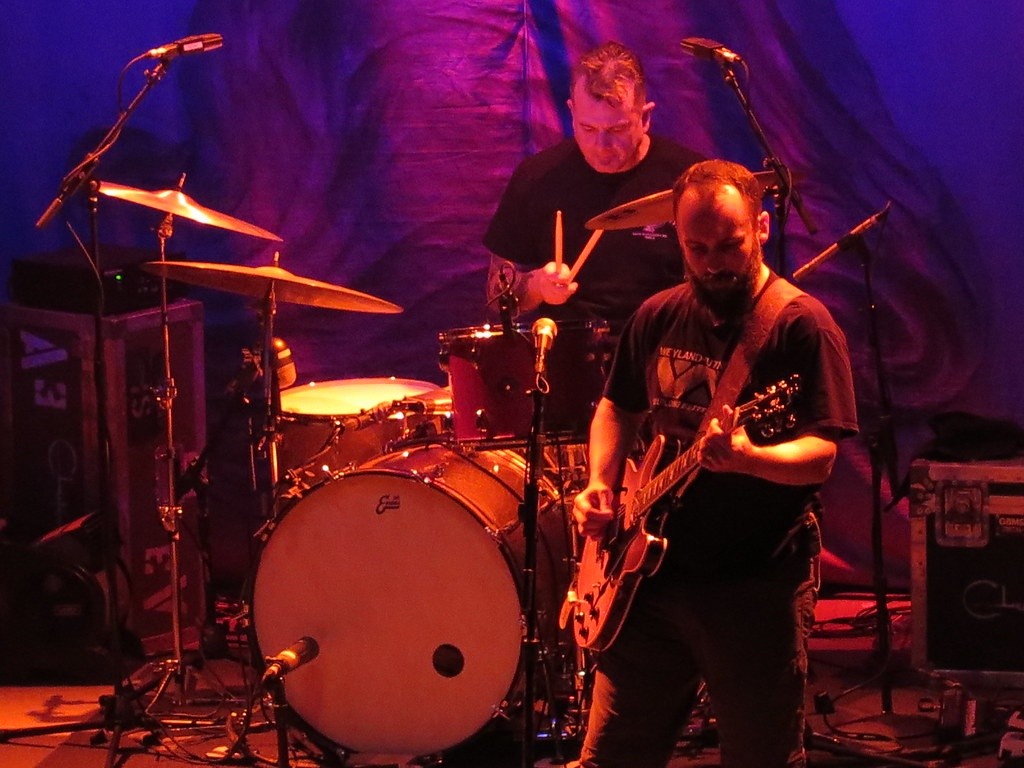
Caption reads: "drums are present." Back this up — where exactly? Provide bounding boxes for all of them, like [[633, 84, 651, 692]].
[[249, 321, 612, 760]]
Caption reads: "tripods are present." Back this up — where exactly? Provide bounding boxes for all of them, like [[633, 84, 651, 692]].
[[0, 61, 261, 768]]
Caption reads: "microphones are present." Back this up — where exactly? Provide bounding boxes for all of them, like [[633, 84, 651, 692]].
[[497, 271, 520, 320], [339, 400, 395, 434], [255, 312, 297, 390], [264, 636, 320, 680], [532, 318, 558, 374], [792, 213, 887, 283], [680, 37, 742, 65], [140, 34, 224, 62]]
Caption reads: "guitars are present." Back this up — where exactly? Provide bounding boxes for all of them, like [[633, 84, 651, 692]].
[[569, 371, 811, 653]]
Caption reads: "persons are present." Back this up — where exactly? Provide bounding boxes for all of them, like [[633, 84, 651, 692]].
[[574, 160, 860, 768], [482, 41, 708, 433]]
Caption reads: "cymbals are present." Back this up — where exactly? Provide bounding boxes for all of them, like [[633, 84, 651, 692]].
[[145, 260, 405, 318], [89, 179, 285, 240], [584, 167, 784, 238]]
